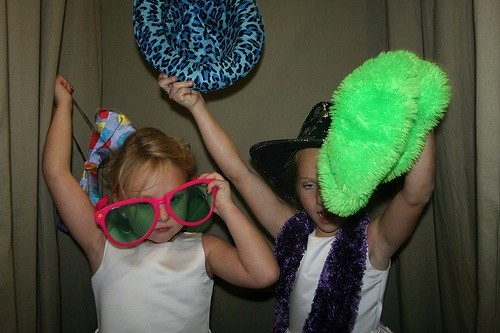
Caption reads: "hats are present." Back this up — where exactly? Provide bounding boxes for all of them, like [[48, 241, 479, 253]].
[[133, 0, 265, 93], [250, 100, 330, 207], [316, 50, 451, 217]]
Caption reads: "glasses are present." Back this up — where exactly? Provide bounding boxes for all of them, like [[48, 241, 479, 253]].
[[95, 178, 217, 246]]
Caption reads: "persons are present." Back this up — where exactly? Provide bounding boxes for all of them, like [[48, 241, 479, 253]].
[[157, 72, 437, 333], [40, 74, 281, 332]]
